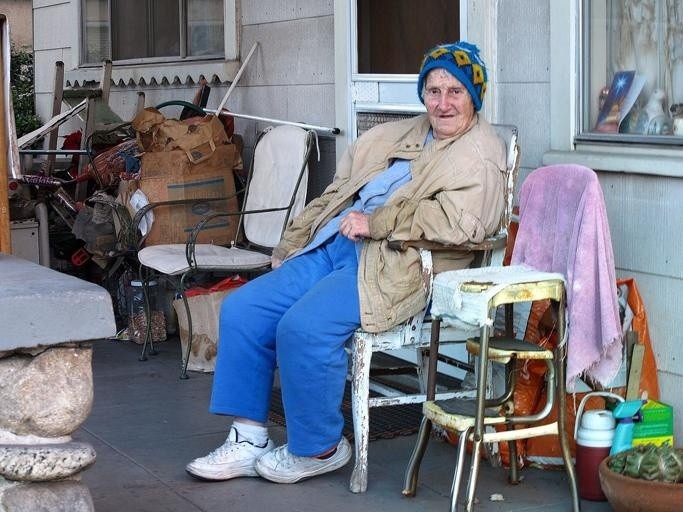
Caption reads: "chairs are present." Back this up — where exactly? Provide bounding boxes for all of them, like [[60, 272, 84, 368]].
[[345, 122, 528, 493], [135, 123, 314, 379], [402, 162, 603, 511], [1, 12, 212, 207]]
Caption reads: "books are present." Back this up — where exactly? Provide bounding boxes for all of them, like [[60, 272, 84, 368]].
[[591, 68, 646, 134]]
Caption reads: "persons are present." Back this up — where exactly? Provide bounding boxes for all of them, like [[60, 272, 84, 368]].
[[184, 39, 507, 484]]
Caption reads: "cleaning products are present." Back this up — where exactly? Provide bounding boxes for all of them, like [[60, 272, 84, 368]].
[[610, 400, 648, 457]]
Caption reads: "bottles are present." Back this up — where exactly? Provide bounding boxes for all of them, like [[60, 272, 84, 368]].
[[127, 281, 168, 344]]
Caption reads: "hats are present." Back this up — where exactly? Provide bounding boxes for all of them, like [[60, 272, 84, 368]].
[[418, 41, 489, 111]]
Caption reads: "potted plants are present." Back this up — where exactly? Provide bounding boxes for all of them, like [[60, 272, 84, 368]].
[[598, 441, 683, 512]]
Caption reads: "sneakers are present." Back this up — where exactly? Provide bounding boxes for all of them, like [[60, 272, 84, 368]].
[[185, 425, 276, 481], [254, 434, 354, 485]]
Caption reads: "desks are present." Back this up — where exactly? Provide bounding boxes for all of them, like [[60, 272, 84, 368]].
[[0, 248, 120, 512]]
